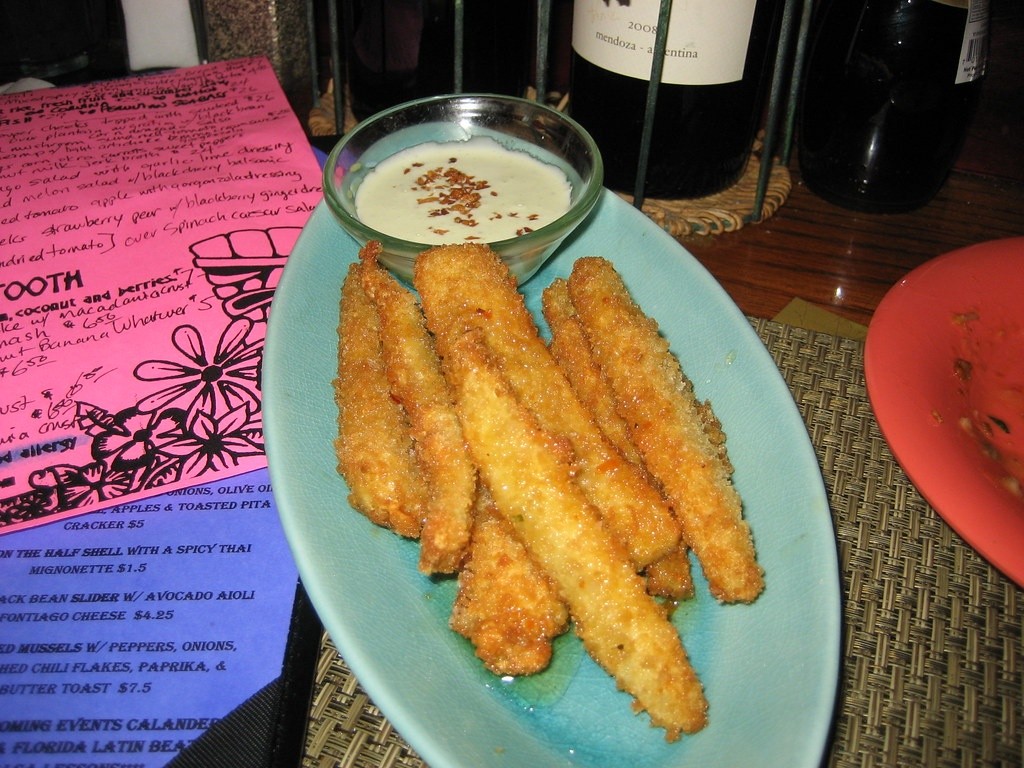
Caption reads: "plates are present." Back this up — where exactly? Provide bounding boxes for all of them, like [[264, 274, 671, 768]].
[[864, 237, 1024, 589], [261, 175, 844, 768]]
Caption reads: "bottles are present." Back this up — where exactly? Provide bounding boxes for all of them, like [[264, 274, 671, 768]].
[[563, 0, 785, 200], [796, 1, 998, 218]]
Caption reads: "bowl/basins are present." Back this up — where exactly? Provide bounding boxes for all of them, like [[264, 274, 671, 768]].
[[324, 90, 605, 297]]
